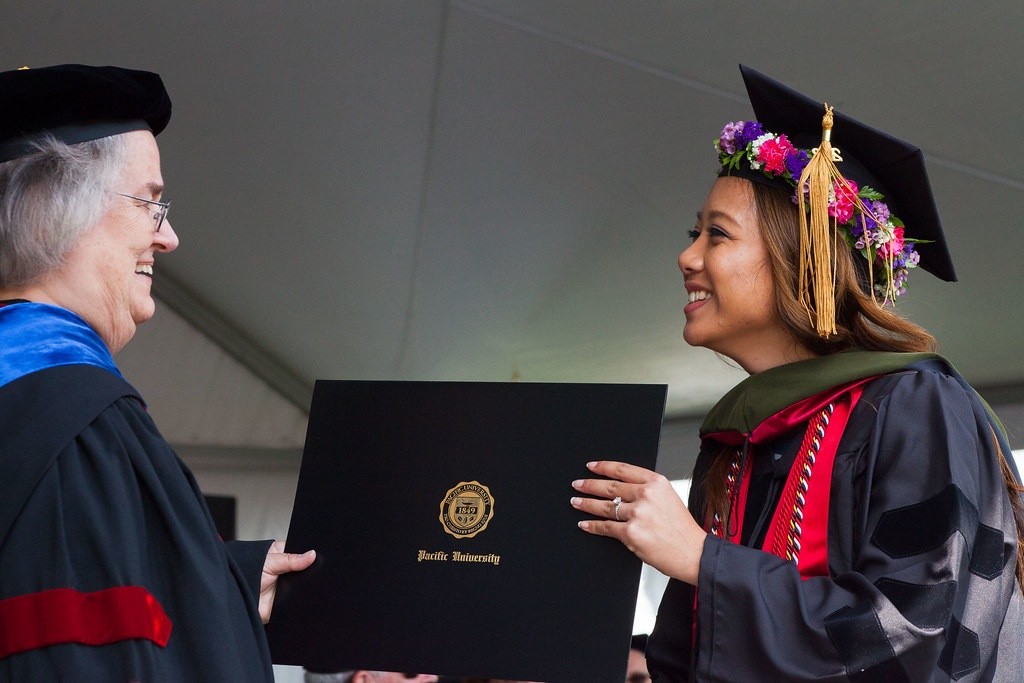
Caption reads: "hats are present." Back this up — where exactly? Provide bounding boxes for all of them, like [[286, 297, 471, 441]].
[[719, 64, 959, 339], [0, 64, 172, 163]]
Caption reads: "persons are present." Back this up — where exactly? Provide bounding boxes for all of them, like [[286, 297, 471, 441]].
[[571, 63, 1024, 683], [0, 66, 316, 683], [626, 633, 652, 683], [300, 665, 439, 683]]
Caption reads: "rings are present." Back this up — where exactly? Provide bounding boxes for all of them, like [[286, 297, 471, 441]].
[[613, 497, 624, 521]]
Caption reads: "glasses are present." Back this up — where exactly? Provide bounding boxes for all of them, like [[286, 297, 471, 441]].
[[103, 189, 172, 233]]
